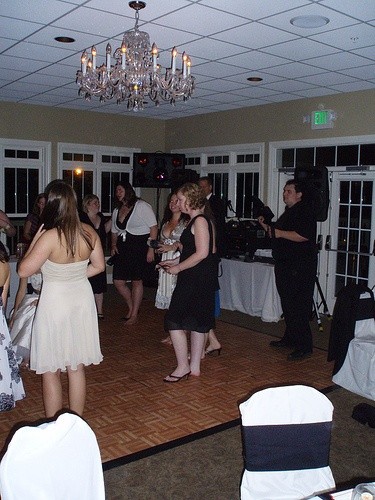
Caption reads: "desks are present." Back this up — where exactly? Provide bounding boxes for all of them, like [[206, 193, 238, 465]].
[[5, 255, 42, 318], [219, 255, 287, 323]]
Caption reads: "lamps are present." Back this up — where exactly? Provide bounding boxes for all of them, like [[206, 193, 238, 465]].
[[303, 109, 337, 130], [76, 2, 196, 113]]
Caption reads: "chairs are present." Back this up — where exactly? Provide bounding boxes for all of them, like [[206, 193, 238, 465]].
[[0, 408, 104, 500], [331, 282, 374, 400], [240, 385, 336, 500]]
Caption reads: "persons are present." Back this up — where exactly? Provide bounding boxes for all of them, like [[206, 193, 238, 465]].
[[163, 182, 221, 383], [154, 190, 191, 345], [198, 177, 224, 235], [0, 210, 27, 411], [80, 193, 112, 325], [25, 194, 47, 241], [111, 181, 158, 326], [187, 199, 222, 360], [258, 180, 318, 360], [17, 179, 107, 422]]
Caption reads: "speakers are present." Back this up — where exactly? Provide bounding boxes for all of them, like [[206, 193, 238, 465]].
[[294, 165, 329, 221]]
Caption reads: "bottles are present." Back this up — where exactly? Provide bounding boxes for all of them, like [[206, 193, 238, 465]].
[[16, 243, 26, 260]]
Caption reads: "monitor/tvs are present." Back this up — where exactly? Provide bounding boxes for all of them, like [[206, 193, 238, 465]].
[[132, 152, 185, 189]]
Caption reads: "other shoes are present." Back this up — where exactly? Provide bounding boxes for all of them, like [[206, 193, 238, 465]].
[[121, 315, 129, 321], [287, 349, 306, 358], [270, 336, 298, 347], [98, 313, 104, 320], [19, 359, 30, 369]]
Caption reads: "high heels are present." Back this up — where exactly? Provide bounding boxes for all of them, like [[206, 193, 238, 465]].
[[204, 346, 222, 356], [163, 371, 192, 382]]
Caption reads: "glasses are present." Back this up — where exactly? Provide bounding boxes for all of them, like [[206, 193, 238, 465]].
[[40, 202, 46, 205]]
